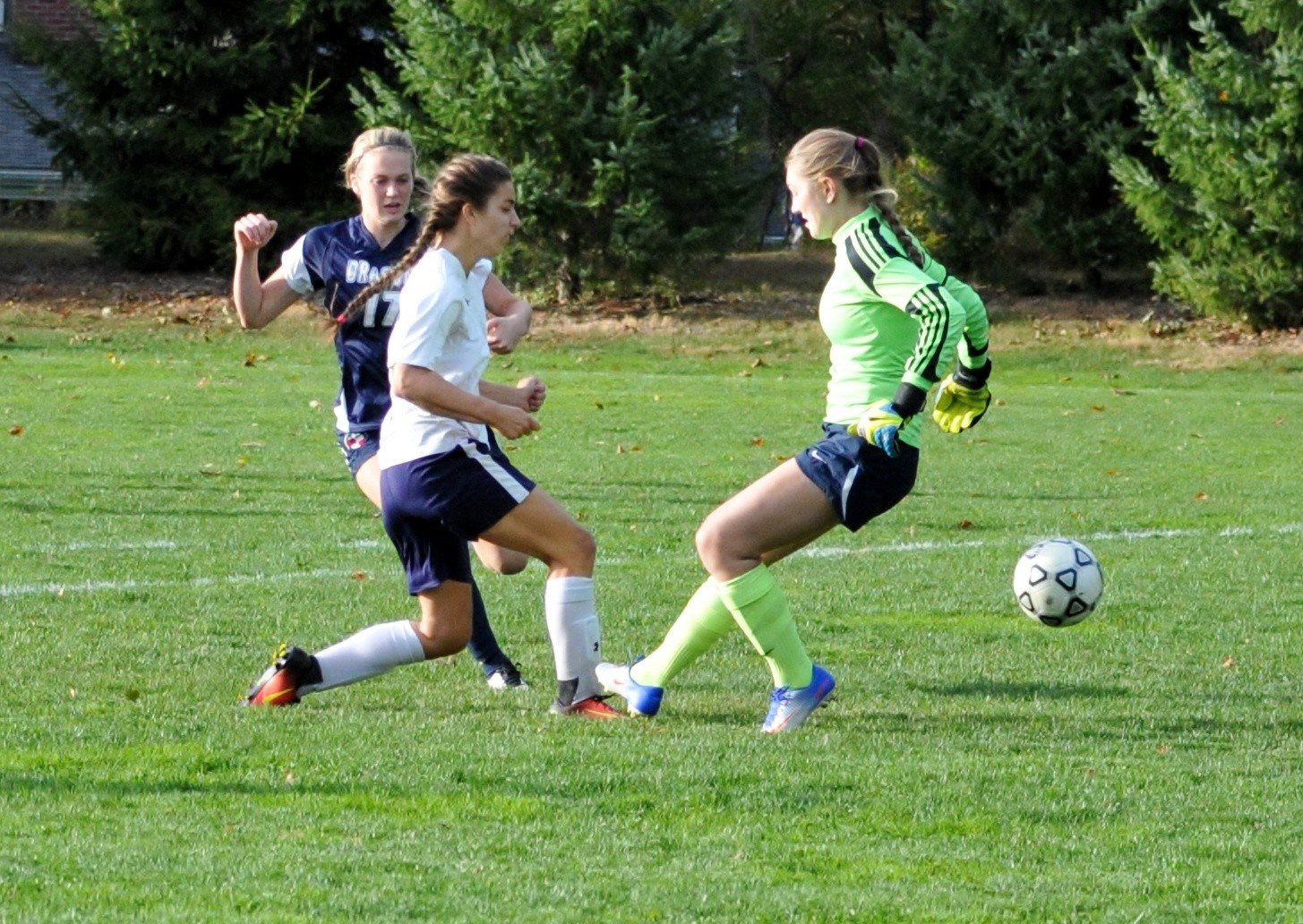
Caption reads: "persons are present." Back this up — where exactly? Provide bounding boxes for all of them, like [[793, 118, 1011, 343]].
[[235, 125, 534, 695], [591, 126, 1000, 741], [240, 148, 631, 717]]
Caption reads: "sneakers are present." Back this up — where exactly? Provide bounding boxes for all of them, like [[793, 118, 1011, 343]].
[[237, 643, 311, 708], [595, 657, 663, 719], [549, 696, 628, 720], [761, 665, 835, 736], [487, 668, 530, 690]]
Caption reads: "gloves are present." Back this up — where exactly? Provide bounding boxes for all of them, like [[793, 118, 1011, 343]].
[[931, 375, 993, 432], [848, 399, 915, 458]]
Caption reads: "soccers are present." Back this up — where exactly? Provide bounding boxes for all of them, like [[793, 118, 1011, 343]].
[[1010, 536, 1105, 628]]
